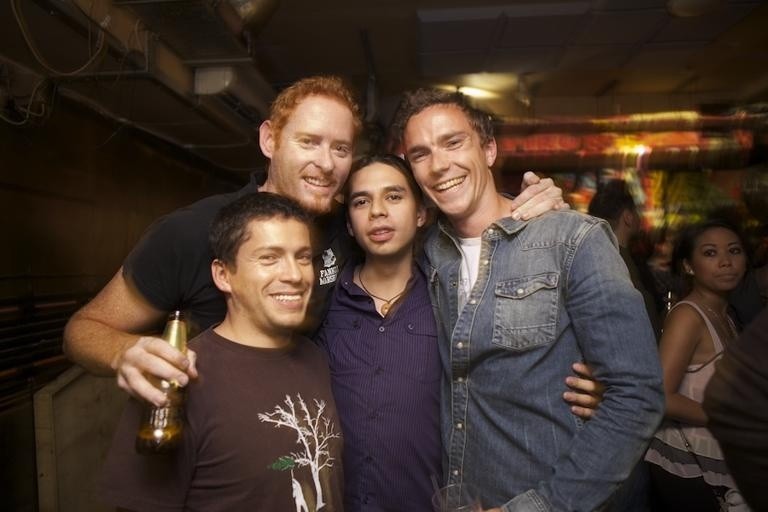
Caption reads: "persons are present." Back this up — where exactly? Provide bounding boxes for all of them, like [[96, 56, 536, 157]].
[[313, 150, 602, 512], [702, 302, 767, 509], [62, 74, 571, 409], [587, 182, 658, 320], [93, 192, 346, 512], [643, 214, 752, 512], [394, 82, 663, 512]]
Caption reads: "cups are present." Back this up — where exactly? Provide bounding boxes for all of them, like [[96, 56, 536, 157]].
[[430, 481, 483, 512]]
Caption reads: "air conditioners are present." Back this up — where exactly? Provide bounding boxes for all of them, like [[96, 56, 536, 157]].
[[193, 56, 272, 130]]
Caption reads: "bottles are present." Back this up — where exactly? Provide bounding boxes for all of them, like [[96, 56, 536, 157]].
[[132, 309, 188, 459], [662, 287, 678, 317]]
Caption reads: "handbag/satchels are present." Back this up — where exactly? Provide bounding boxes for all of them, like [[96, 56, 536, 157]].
[[717, 487, 753, 511]]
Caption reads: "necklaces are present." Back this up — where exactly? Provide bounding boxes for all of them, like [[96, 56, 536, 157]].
[[359, 265, 404, 317], [700, 298, 731, 339]]
